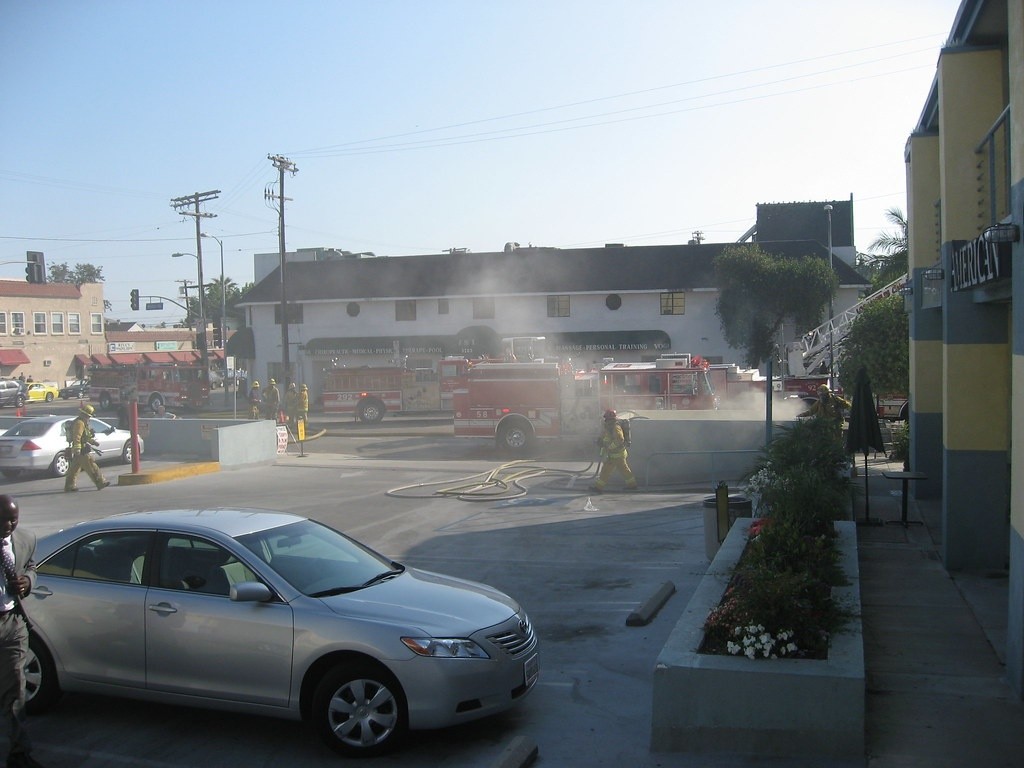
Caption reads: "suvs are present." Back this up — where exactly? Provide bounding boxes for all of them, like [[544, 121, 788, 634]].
[[877, 396, 910, 421], [0, 381, 30, 408]]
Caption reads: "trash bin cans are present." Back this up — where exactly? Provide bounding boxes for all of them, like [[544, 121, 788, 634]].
[[703, 498, 752, 561]]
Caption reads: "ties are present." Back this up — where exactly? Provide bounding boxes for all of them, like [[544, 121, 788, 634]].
[[0, 539, 18, 596]]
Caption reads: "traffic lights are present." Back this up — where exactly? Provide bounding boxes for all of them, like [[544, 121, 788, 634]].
[[131, 289, 139, 312]]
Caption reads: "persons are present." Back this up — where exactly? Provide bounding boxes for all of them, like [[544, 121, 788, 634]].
[[589, 409, 640, 494], [13, 372, 33, 382], [818, 361, 827, 373], [64, 405, 111, 492], [797, 384, 852, 438], [248, 379, 308, 435], [0, 494, 45, 768]]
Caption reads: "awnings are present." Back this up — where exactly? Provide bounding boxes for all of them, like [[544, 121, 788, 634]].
[[0, 348, 31, 366], [76, 351, 234, 366]]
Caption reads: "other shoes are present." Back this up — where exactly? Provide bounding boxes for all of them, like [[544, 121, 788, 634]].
[[97, 481, 111, 491], [6, 753, 45, 768], [588, 486, 601, 495], [64, 486, 79, 492], [623, 485, 638, 492]]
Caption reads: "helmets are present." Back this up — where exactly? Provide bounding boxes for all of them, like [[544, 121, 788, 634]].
[[301, 384, 309, 391], [79, 404, 94, 417], [268, 378, 276, 385], [603, 410, 619, 422], [816, 384, 832, 395], [251, 381, 260, 388], [287, 383, 296, 390]]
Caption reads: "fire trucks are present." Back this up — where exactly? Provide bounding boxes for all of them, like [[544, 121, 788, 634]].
[[87, 363, 211, 413], [322, 356, 476, 425], [453, 359, 720, 454], [660, 273, 909, 406]]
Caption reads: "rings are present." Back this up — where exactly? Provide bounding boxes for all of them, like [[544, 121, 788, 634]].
[[23, 588, 24, 592]]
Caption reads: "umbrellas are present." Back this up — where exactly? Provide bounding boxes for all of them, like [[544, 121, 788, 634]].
[[846, 363, 886, 519]]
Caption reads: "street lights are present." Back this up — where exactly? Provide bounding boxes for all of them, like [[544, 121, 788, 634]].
[[824, 204, 834, 391], [200, 232, 231, 406], [173, 254, 203, 319]]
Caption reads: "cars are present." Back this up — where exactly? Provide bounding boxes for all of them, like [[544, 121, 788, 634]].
[[24, 382, 59, 402], [22, 507, 543, 750], [0, 413, 144, 481], [59, 377, 91, 400]]
